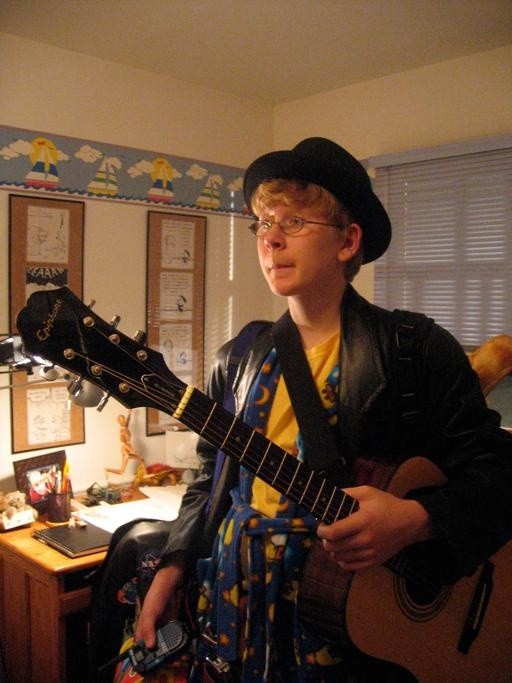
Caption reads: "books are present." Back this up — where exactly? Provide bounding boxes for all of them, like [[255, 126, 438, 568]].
[[32, 521, 112, 557]]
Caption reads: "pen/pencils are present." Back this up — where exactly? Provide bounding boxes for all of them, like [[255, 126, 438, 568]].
[[51, 462, 71, 493]]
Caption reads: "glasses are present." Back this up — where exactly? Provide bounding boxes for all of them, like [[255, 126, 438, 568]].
[[248, 217, 343, 237]]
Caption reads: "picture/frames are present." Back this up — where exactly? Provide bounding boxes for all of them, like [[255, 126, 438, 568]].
[[12, 449, 74, 514], [6, 193, 85, 453], [145, 210, 207, 436]]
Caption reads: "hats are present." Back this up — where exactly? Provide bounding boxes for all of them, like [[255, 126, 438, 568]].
[[241, 134, 392, 267]]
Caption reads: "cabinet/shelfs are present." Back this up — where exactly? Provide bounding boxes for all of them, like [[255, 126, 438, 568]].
[[0, 469, 202, 683]]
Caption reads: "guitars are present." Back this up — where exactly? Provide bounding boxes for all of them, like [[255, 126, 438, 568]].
[[0, 281, 510, 682]]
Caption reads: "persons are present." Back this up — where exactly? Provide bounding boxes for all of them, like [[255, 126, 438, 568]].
[[102, 409, 147, 478], [29, 471, 50, 501], [132, 136, 512, 683]]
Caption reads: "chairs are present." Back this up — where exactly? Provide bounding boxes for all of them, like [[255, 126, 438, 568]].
[[63, 520, 179, 683]]
